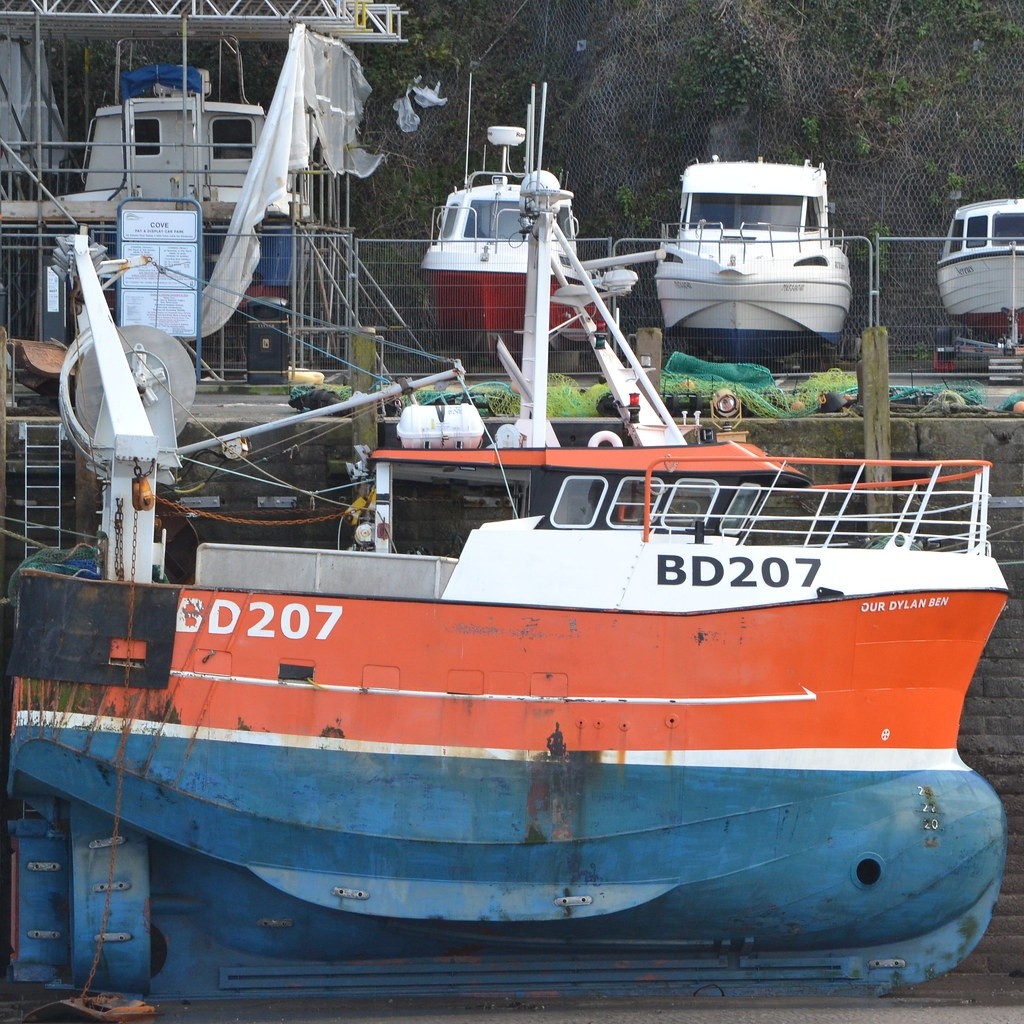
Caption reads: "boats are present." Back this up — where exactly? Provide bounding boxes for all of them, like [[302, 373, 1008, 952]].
[[937, 199, 1024, 339], [0, 27, 307, 383], [651, 157, 852, 362], [7, 82, 1005, 998], [417, 71, 610, 360]]
